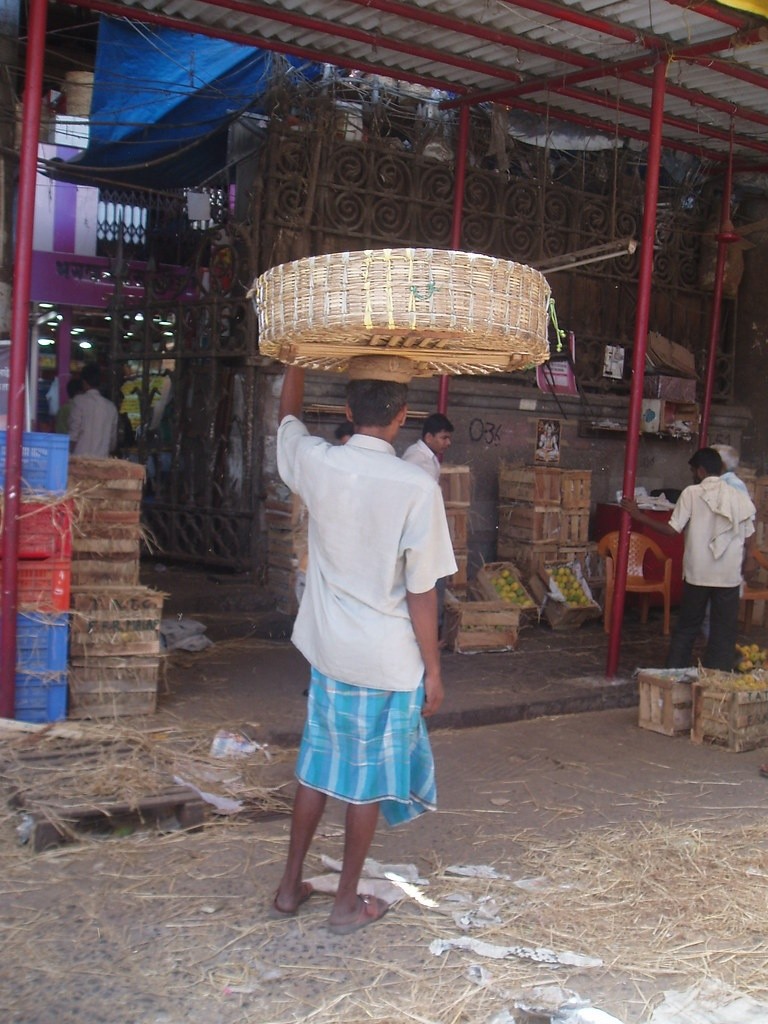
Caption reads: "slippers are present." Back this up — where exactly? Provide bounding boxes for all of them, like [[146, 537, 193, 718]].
[[272, 880, 314, 916], [328, 893, 389, 934]]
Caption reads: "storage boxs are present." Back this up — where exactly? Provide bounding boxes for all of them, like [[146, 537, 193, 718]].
[[644, 374, 696, 403], [1, 431, 163, 722], [639, 665, 768, 752], [736, 474, 768, 626], [47, 115, 89, 148], [438, 463, 607, 655], [264, 483, 309, 617]]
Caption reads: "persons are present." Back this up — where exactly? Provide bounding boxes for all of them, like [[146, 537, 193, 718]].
[[275, 355, 460, 936], [696, 444, 752, 669], [621, 447, 756, 675], [54, 375, 135, 461]]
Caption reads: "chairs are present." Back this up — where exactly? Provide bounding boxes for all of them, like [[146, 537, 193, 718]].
[[597, 530, 672, 635], [740, 543, 768, 633]]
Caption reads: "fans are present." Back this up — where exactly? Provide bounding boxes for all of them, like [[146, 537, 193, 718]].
[[655, 114, 768, 250]]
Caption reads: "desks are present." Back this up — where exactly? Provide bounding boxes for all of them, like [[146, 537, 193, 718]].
[[595, 500, 685, 621]]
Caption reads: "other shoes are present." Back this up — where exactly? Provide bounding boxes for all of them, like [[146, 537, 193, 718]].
[[760, 762, 768, 778]]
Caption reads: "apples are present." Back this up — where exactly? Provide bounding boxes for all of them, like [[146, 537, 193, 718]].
[[735, 643, 768, 671], [545, 566, 589, 607], [490, 570, 531, 606]]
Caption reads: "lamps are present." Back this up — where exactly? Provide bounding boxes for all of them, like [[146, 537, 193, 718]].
[[524, 71, 637, 274]]
